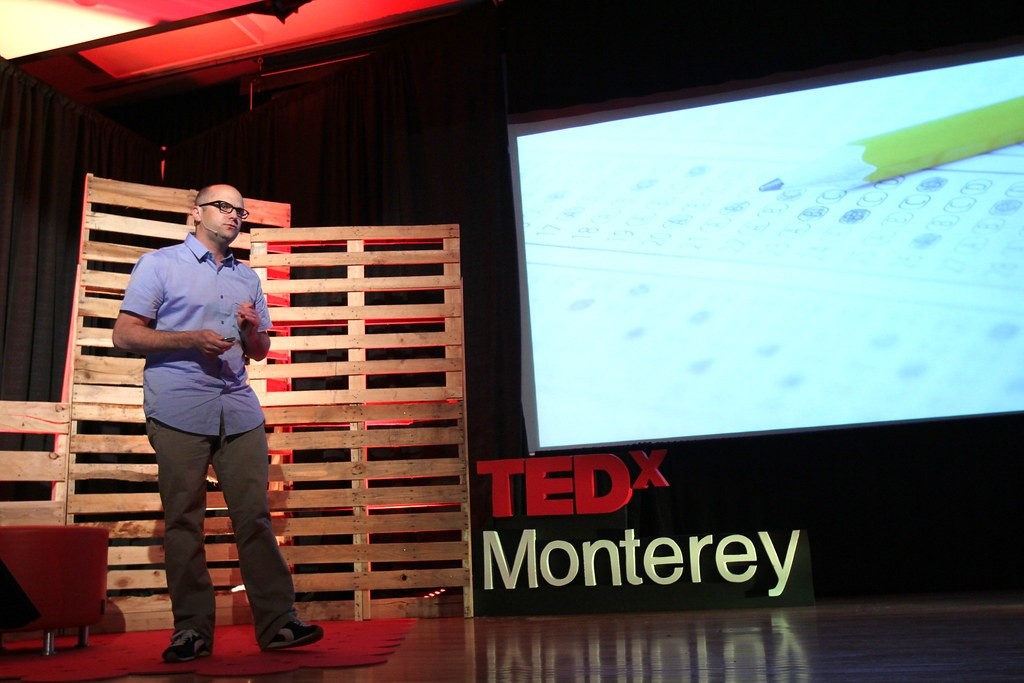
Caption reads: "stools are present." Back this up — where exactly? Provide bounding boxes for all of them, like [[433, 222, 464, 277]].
[[0, 525, 109, 657]]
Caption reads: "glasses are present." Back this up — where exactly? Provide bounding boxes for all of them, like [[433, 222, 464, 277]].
[[198, 200, 250, 220]]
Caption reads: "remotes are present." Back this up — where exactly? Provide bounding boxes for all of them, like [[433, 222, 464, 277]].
[[224, 337, 236, 342]]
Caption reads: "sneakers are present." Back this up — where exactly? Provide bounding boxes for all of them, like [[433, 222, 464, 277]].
[[261, 616, 323, 653], [162, 629, 213, 663]]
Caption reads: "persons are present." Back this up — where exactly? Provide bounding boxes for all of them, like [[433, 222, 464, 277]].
[[112, 183, 324, 664]]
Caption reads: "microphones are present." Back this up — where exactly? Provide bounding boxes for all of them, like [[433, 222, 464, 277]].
[[202, 220, 218, 234]]
